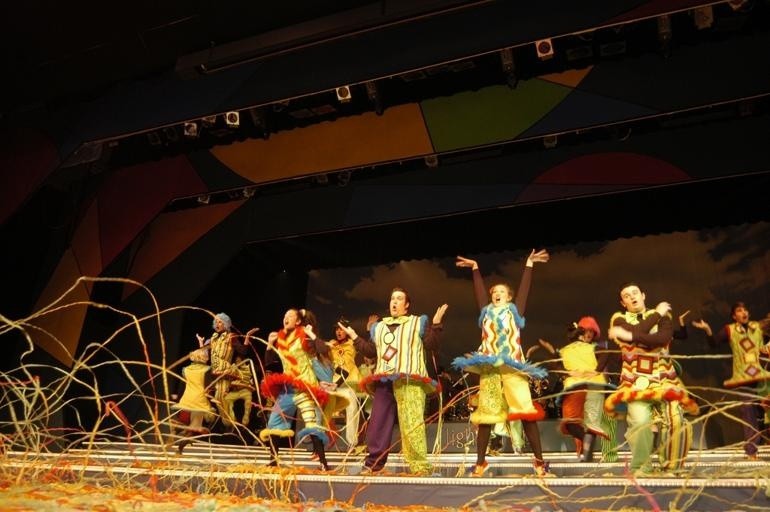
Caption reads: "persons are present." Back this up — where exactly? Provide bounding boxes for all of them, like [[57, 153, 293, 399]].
[[691, 300, 770, 461], [450, 250, 699, 475], [172, 288, 450, 477]]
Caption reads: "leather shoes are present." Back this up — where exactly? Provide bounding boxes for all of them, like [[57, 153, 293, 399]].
[[578, 453, 592, 463]]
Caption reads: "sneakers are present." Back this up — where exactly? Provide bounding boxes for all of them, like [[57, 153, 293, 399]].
[[532, 462, 557, 479], [319, 460, 328, 472], [747, 452, 758, 462], [468, 459, 490, 478], [357, 466, 372, 475]]
